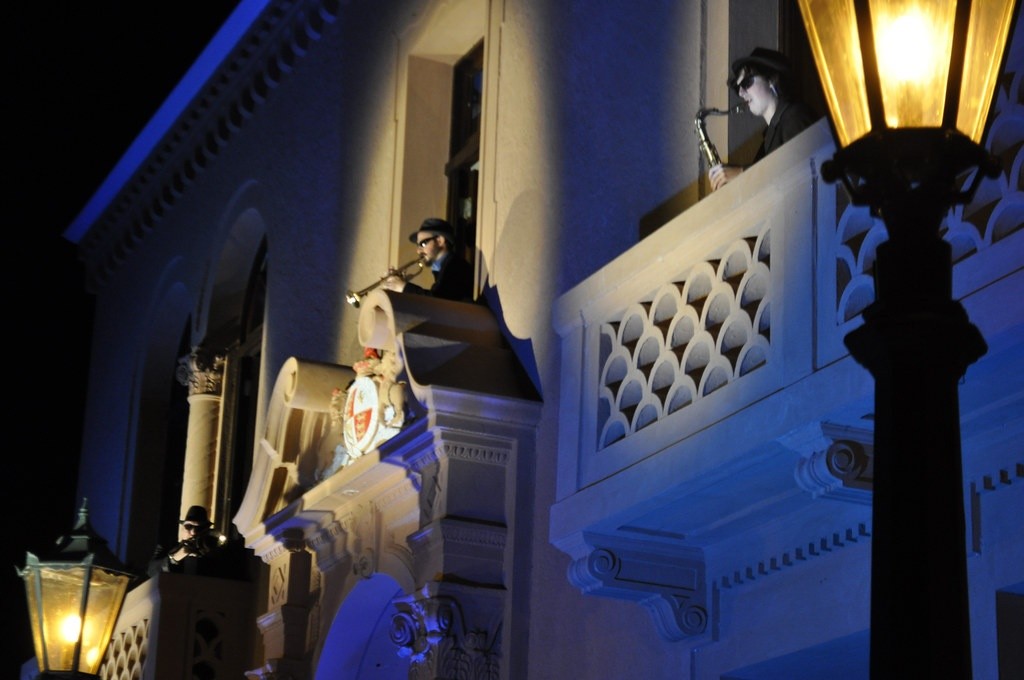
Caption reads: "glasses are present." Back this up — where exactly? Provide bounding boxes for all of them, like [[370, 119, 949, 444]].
[[420, 236, 439, 248], [734, 73, 761, 93], [185, 525, 204, 533]]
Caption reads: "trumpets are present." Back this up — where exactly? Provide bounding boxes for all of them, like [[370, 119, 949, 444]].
[[345, 255, 424, 309]]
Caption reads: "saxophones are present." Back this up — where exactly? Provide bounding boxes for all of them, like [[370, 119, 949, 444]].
[[692, 101, 749, 185]]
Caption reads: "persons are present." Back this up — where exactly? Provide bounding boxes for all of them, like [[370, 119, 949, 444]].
[[386, 218, 474, 302], [709, 49, 818, 190], [147, 506, 234, 581]]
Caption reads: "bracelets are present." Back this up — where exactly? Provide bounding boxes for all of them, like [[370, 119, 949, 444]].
[[169, 555, 178, 565]]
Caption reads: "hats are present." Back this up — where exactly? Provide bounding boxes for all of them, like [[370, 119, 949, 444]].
[[408, 218, 456, 253], [177, 506, 212, 529], [731, 47, 795, 81]]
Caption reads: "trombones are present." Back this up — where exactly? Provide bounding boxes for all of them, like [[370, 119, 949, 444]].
[[167, 529, 229, 565]]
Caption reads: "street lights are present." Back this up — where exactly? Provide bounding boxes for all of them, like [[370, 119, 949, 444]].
[[797, 1, 1018, 680]]
